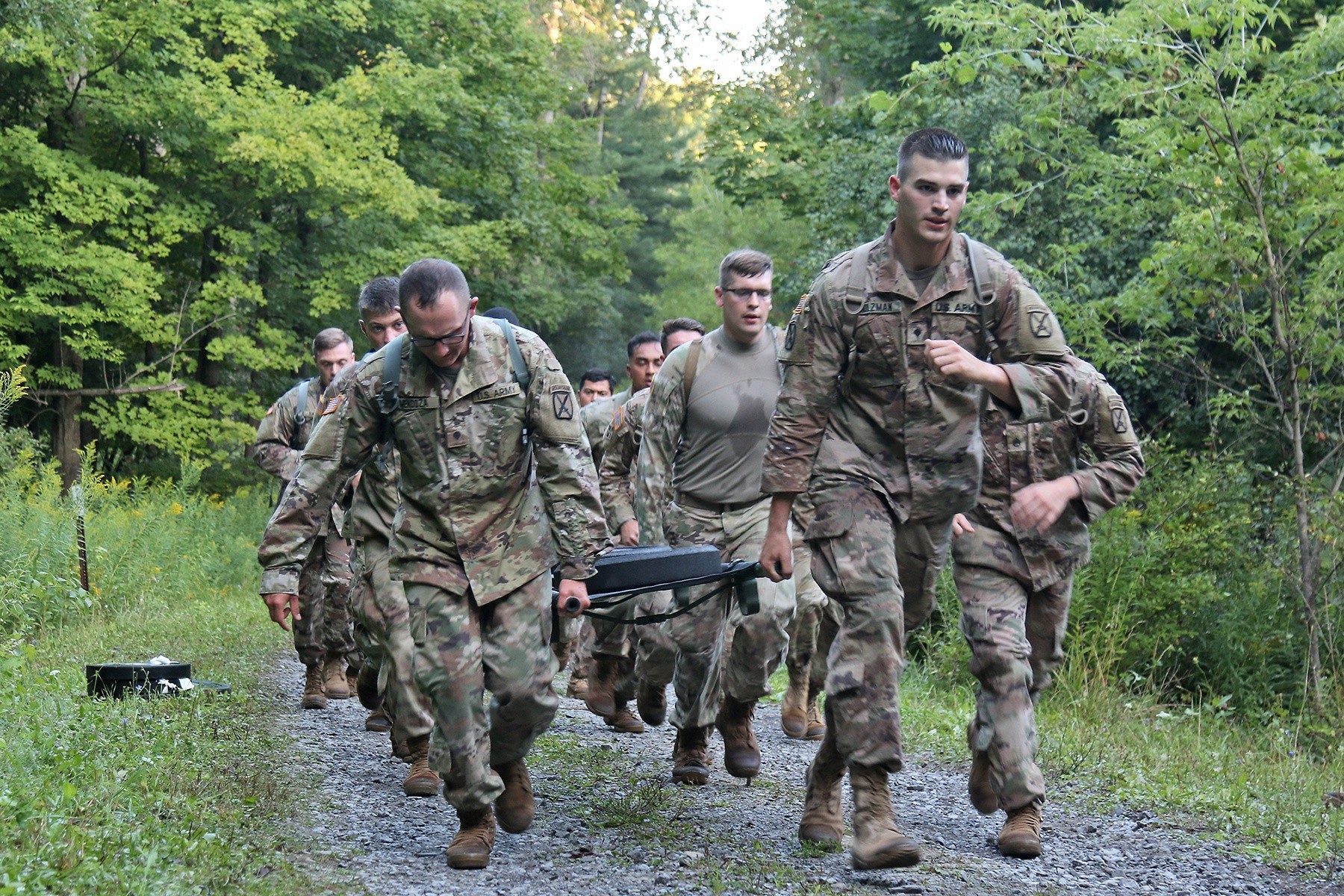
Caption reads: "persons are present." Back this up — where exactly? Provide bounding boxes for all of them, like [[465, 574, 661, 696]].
[[257, 257, 610, 870], [757, 126, 1078, 873], [951, 333, 1147, 860], [253, 248, 827, 798]]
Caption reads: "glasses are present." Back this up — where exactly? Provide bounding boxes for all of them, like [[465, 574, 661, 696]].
[[400, 297, 472, 348], [722, 287, 772, 301]]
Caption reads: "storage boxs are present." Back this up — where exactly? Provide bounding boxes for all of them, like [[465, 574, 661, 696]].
[[86, 662, 192, 701]]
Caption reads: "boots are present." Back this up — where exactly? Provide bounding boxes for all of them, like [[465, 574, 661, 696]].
[[798, 754, 843, 850], [638, 680, 666, 726], [565, 666, 589, 699], [301, 663, 326, 709], [365, 709, 392, 731], [967, 720, 999, 815], [781, 658, 828, 740], [321, 657, 350, 698], [997, 792, 1046, 860], [604, 702, 645, 732], [587, 658, 615, 718], [672, 727, 709, 784], [447, 803, 496, 869], [390, 728, 409, 758], [403, 732, 441, 795], [492, 759, 535, 834], [345, 664, 359, 697], [851, 774, 919, 870], [715, 696, 760, 778]]
